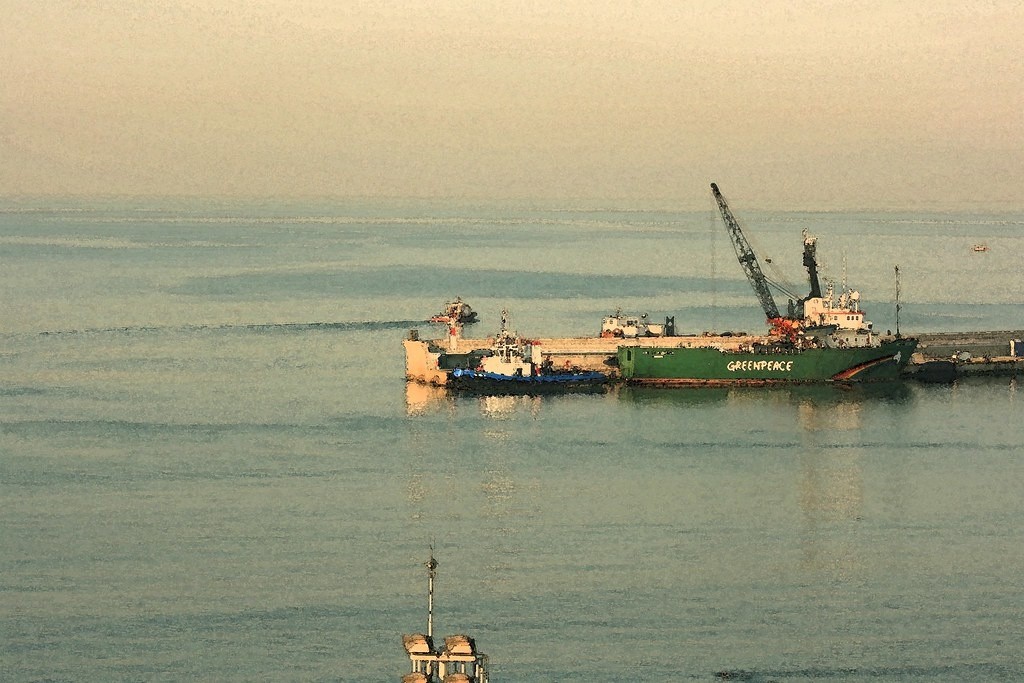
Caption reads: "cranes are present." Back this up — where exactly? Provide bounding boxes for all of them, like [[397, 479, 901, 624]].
[[711, 181, 807, 334]]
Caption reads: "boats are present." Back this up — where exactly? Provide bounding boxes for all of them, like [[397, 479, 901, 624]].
[[396, 535, 491, 683], [615, 226, 919, 395], [444, 342, 609, 393]]
